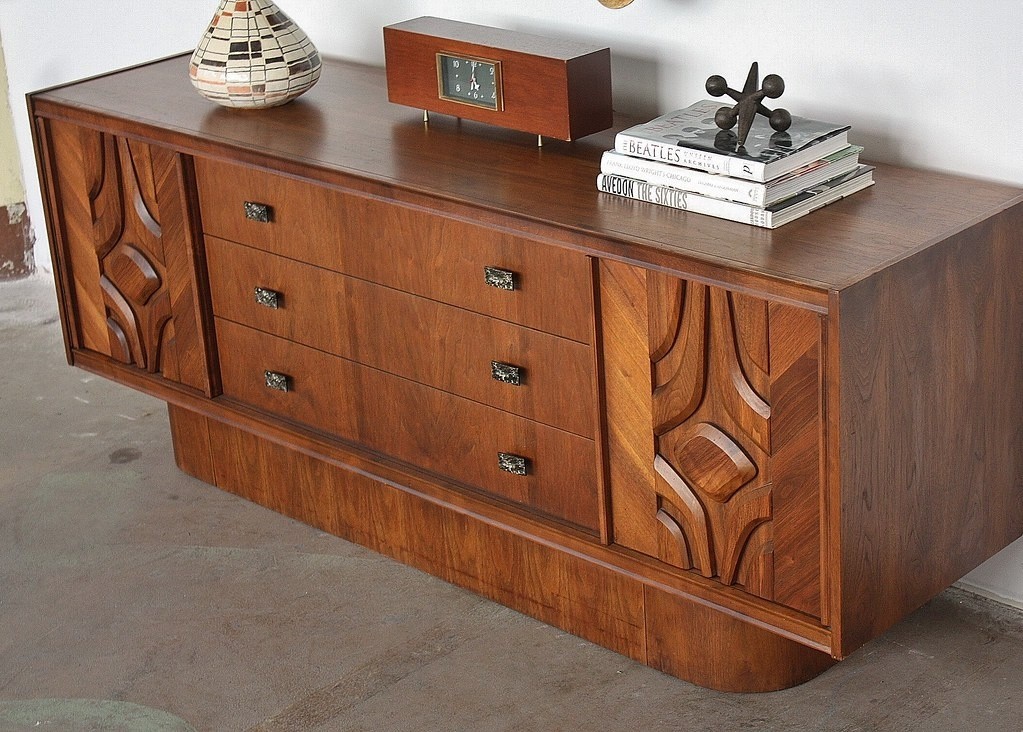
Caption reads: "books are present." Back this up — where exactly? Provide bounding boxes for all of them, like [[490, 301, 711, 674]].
[[596, 99, 877, 230]]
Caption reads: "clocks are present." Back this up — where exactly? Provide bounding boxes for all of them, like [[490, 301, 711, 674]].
[[382, 14, 613, 146]]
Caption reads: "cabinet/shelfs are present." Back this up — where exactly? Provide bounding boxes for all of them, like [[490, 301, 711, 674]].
[[23, 51, 1023, 698]]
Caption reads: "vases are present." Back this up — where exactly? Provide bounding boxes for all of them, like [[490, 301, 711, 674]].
[[188, 0, 321, 109]]
